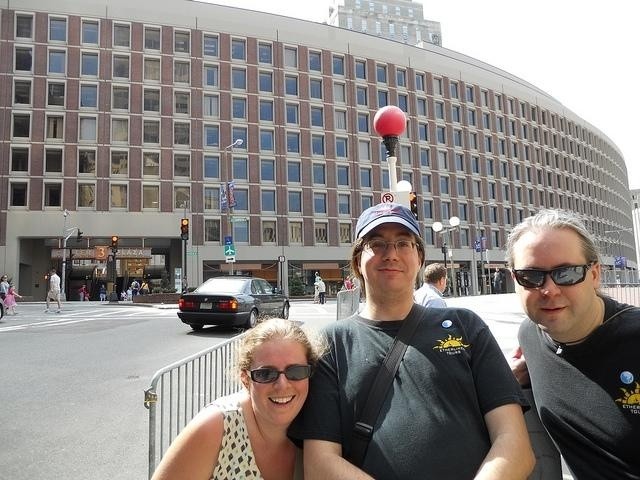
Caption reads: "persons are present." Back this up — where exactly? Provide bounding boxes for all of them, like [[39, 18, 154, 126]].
[[492, 267, 504, 294], [413, 262, 448, 309], [286, 203, 537, 479], [343, 273, 363, 303], [0, 274, 22, 316], [149, 318, 333, 480], [85, 293, 90, 301], [121, 277, 149, 301], [44, 268, 61, 313], [314, 271, 326, 304], [78, 285, 86, 301], [504, 206, 640, 480], [99, 284, 106, 301]]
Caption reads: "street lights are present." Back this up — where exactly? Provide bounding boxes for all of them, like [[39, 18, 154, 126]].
[[62, 208, 70, 302], [223, 139, 245, 276], [372, 103, 409, 207], [605, 227, 633, 285], [433, 215, 461, 295], [477, 202, 494, 294]]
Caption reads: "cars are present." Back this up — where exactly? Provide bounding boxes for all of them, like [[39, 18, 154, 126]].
[[0, 297, 5, 321], [177, 276, 292, 331]]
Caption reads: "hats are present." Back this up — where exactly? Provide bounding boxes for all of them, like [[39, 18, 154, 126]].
[[355, 203, 420, 241]]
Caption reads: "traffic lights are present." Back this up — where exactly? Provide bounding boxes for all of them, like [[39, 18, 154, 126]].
[[78, 230, 83, 242], [112, 236, 118, 253], [181, 219, 189, 240]]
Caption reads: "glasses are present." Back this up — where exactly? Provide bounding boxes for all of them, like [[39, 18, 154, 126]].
[[512, 260, 597, 289], [246, 364, 312, 385], [363, 238, 422, 255]]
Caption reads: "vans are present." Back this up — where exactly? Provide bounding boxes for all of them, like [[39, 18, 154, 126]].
[[294, 282, 315, 296]]
